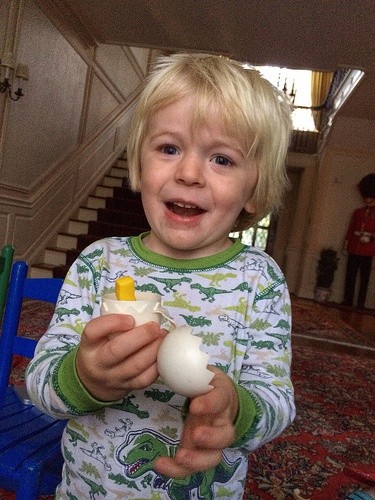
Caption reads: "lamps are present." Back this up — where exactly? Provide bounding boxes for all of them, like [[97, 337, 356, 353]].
[[0, 51, 30, 101]]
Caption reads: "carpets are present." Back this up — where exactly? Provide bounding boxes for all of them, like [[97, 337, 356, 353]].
[[52, 177, 148, 279]]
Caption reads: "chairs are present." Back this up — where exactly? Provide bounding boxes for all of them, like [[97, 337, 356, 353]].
[[0, 260, 68, 500]]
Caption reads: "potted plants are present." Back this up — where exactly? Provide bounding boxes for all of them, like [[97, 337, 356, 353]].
[[313, 247, 340, 303]]
